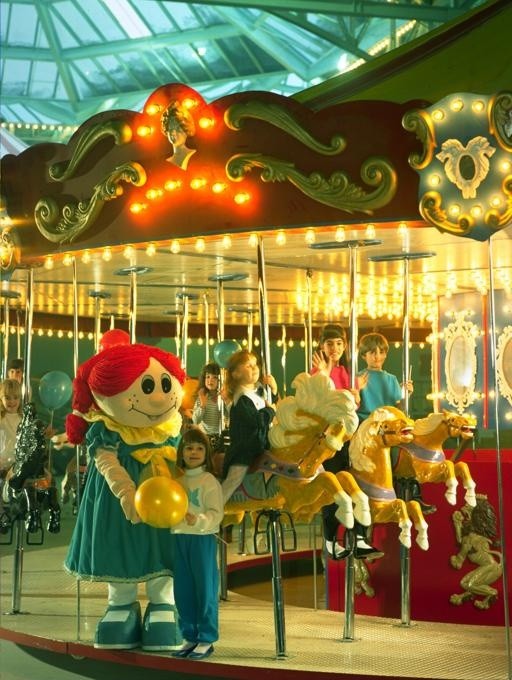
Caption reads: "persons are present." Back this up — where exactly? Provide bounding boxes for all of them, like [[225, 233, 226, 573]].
[[7, 358, 32, 401], [222, 350, 279, 505], [191, 364, 223, 451], [159, 101, 197, 171], [357, 331, 438, 516], [306, 323, 384, 562], [0, 378, 53, 524], [62, 342, 189, 652], [169, 430, 224, 658]]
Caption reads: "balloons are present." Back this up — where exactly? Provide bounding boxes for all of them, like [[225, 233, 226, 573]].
[[98, 329, 130, 353], [214, 339, 242, 371], [183, 379, 199, 409], [38, 369, 72, 412], [133, 476, 189, 529]]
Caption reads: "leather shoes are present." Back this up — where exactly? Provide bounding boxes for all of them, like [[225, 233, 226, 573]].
[[170, 638, 197, 661], [352, 536, 385, 560], [185, 640, 217, 661], [323, 540, 353, 562]]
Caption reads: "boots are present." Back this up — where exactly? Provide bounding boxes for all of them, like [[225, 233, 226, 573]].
[[407, 480, 438, 515]]
